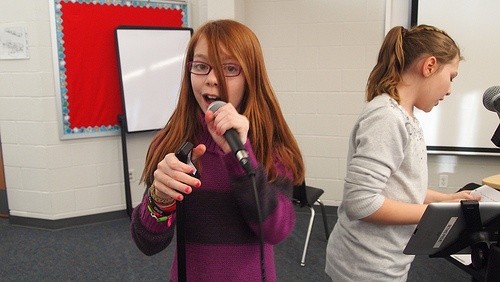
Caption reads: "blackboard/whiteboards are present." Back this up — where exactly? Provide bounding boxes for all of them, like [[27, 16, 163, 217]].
[[412, 0, 500, 157], [112, 24, 197, 135]]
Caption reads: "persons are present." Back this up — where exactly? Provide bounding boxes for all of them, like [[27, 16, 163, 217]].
[[324, 23, 482, 282], [129, 18, 306, 282]]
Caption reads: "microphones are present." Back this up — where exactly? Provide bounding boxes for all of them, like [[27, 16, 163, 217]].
[[207, 101, 249, 166], [483, 86, 500, 119]]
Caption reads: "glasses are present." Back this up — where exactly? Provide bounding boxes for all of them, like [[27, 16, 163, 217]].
[[188, 61, 242, 77]]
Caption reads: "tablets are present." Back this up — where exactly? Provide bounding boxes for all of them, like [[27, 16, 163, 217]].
[[403, 202, 500, 256]]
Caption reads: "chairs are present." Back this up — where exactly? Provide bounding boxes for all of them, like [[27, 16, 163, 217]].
[[291, 179, 329, 266]]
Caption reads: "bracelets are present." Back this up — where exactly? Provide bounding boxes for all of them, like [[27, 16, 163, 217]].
[[146, 183, 178, 228]]
